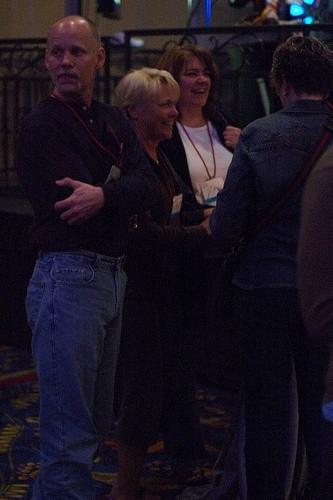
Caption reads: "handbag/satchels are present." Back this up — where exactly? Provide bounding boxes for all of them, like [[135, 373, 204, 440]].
[[207, 237, 245, 324]]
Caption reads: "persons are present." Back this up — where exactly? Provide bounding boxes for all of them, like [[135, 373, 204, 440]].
[[297, 145, 333, 360], [156, 46, 243, 484], [208, 37, 333, 500], [112, 69, 216, 500], [15, 15, 159, 500]]
